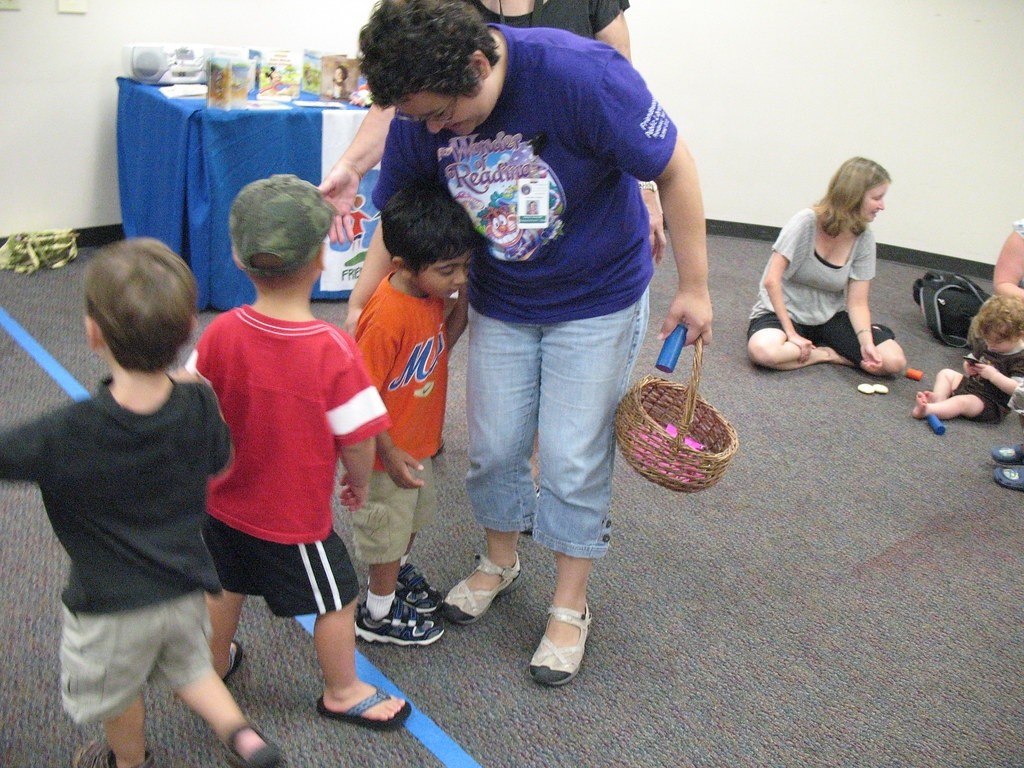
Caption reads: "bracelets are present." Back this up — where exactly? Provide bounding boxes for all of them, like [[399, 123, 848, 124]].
[[856, 329, 872, 337]]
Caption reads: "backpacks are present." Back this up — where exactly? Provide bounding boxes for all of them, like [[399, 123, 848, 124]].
[[913, 271, 991, 349]]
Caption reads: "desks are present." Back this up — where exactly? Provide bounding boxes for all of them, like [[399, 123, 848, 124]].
[[116, 77, 381, 315]]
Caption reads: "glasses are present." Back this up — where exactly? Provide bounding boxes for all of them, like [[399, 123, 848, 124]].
[[394, 87, 461, 124]]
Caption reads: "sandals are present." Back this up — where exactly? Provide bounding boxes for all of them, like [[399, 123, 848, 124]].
[[367, 563, 444, 614], [355, 600, 444, 646], [218, 722, 288, 768], [71, 741, 154, 768]]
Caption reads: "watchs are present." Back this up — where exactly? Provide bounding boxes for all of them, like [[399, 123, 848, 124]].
[[639, 180, 658, 192]]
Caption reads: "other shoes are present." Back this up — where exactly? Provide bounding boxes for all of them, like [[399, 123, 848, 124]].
[[442, 551, 521, 624], [872, 324, 895, 341], [991, 444, 1024, 465], [528, 602, 593, 686], [993, 465, 1024, 492]]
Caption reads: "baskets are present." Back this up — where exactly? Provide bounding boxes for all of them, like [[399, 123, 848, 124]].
[[615, 334, 739, 493]]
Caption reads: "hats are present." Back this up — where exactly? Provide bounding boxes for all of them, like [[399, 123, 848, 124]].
[[229, 174, 338, 278]]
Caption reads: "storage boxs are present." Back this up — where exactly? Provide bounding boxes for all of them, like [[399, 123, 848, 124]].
[[1, 228, 1023, 768]]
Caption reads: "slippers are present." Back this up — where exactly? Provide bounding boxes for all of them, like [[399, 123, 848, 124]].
[[317, 686, 411, 730], [223, 641, 243, 683]]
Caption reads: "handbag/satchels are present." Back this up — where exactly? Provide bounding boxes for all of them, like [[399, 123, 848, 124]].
[[0, 229, 81, 274]]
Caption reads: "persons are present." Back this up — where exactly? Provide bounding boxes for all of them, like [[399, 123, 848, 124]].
[[189, 175, 413, 733], [747, 156, 907, 378], [319, 0, 667, 269], [911, 294, 1024, 424], [344, 1, 715, 689], [992, 217, 1024, 297], [0, 234, 287, 767], [342, 180, 478, 648]]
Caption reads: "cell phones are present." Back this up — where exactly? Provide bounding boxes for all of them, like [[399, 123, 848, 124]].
[[963, 357, 981, 366]]
